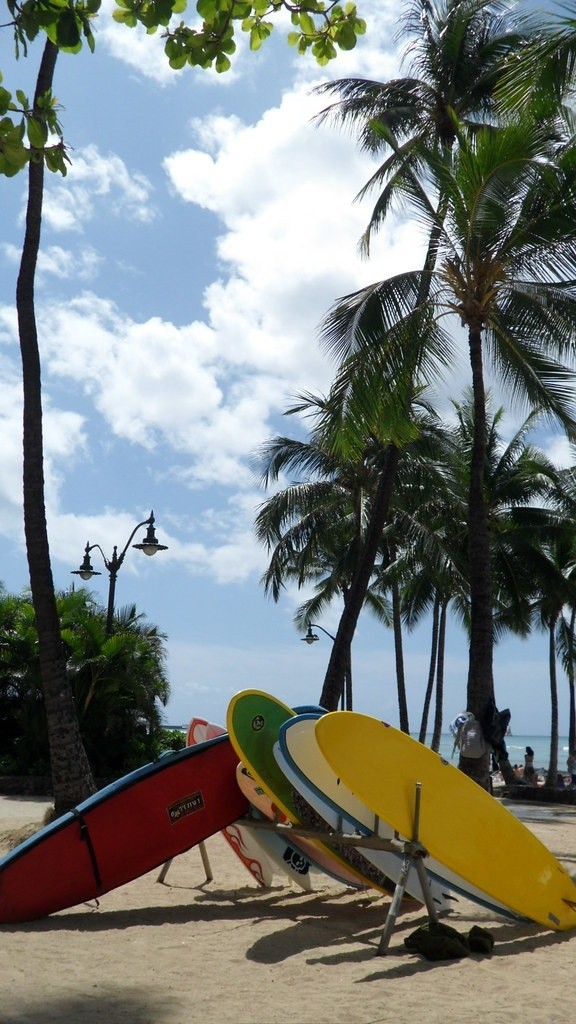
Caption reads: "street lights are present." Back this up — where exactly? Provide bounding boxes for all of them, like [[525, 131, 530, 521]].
[[71, 509, 169, 633], [300, 620, 345, 711]]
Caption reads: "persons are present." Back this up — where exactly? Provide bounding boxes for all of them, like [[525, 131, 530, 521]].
[[524, 746, 538, 786], [567, 750, 576, 787], [510, 763, 571, 784]]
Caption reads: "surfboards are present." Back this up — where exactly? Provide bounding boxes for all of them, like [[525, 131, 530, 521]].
[[0, 704, 330, 924], [186, 688, 576, 932]]
